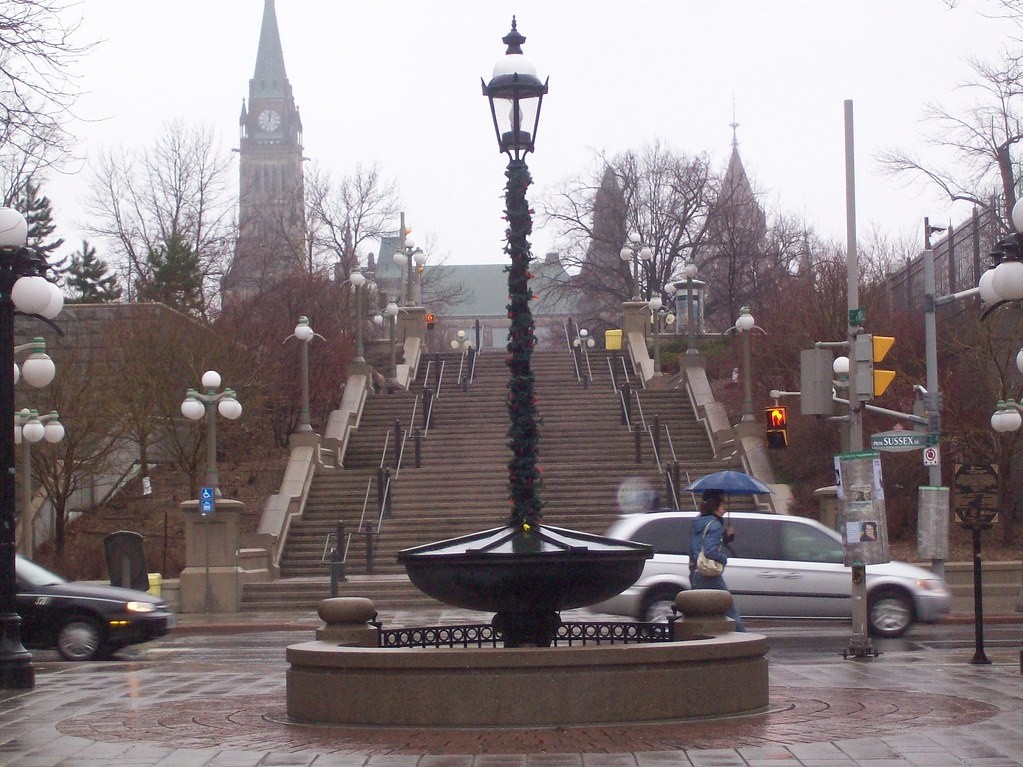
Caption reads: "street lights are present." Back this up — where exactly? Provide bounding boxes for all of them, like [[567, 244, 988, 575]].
[[393, 13, 657, 649], [282, 315, 327, 434], [723, 305, 768, 423], [638, 297, 674, 379], [923, 197, 1023, 576], [339, 267, 377, 364], [374, 298, 410, 379], [664, 257, 701, 355], [180, 371, 242, 496], [620, 232, 652, 301], [0, 207, 65, 690], [393, 244, 428, 307]]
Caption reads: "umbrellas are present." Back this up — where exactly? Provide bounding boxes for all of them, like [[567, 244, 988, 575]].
[[682, 470, 774, 529]]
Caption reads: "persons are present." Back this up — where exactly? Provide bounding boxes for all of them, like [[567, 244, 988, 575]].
[[690, 488, 747, 634]]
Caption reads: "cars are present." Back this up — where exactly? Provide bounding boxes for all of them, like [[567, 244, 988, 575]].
[[583, 512, 953, 639], [15, 550, 173, 660]]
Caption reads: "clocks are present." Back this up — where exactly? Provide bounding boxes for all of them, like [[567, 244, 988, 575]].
[[256, 109, 282, 133]]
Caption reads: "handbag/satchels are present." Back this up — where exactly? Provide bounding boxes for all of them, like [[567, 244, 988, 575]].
[[697, 520, 724, 575]]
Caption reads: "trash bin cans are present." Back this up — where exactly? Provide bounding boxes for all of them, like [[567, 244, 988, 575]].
[[604, 329, 622, 349], [145, 572, 163, 597]]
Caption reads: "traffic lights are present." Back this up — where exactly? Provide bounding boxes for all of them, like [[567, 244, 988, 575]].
[[765, 407, 789, 451], [426, 314, 435, 331], [857, 335, 896, 402]]
